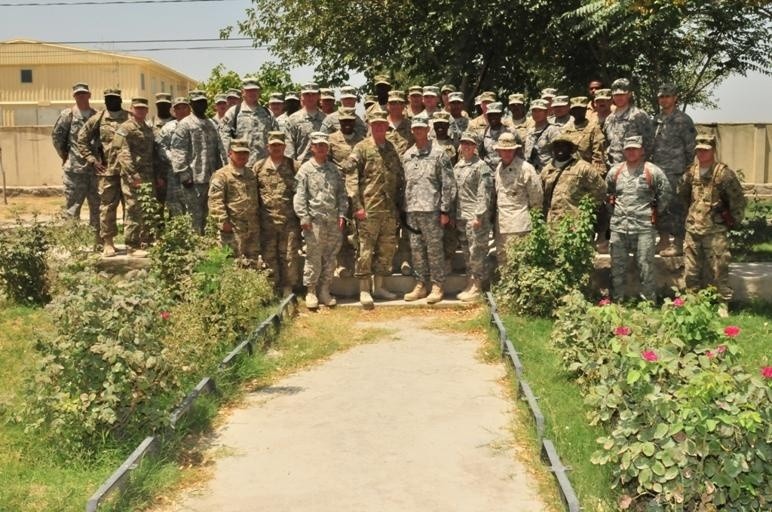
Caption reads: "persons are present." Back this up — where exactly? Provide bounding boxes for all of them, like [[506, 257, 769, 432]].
[[220, 77, 275, 169], [380, 90, 414, 274], [79, 90, 138, 258], [481, 103, 516, 165], [345, 111, 400, 308], [440, 84, 454, 113], [652, 84, 698, 255], [252, 132, 298, 293], [157, 97, 192, 222], [542, 88, 562, 115], [113, 96, 161, 247], [675, 135, 743, 308], [522, 98, 560, 169], [171, 92, 217, 236], [324, 106, 364, 280], [540, 134, 605, 227], [600, 80, 653, 165], [52, 83, 105, 247], [363, 93, 376, 112], [429, 116, 460, 275], [551, 98, 606, 165], [284, 83, 327, 176], [364, 77, 392, 120], [504, 93, 536, 142], [225, 87, 244, 111], [150, 93, 175, 239], [466, 90, 501, 129], [493, 133, 541, 266], [443, 94, 470, 146], [207, 138, 260, 265], [211, 95, 229, 129], [267, 92, 287, 120], [590, 86, 614, 126], [273, 96, 301, 135], [293, 133, 348, 309], [362, 91, 385, 122], [587, 76, 604, 122], [404, 116, 454, 303], [322, 86, 366, 134], [539, 96, 580, 163], [603, 137, 672, 304], [450, 132, 493, 300], [404, 85, 423, 121], [408, 82, 458, 141], [320, 91, 336, 118]]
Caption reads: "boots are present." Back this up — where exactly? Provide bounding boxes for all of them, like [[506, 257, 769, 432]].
[[302, 244, 307, 253], [359, 279, 374, 305], [422, 288, 425, 289], [373, 275, 397, 299], [718, 302, 729, 317], [127, 245, 149, 258], [426, 283, 444, 303], [445, 260, 452, 276], [461, 282, 481, 301], [594, 232, 605, 244], [385, 267, 394, 276], [103, 237, 115, 257], [596, 240, 609, 254], [336, 266, 353, 278], [660, 238, 683, 257], [283, 287, 293, 298], [403, 281, 426, 300], [401, 257, 412, 276], [319, 284, 336, 305], [306, 285, 318, 308], [655, 238, 671, 254], [456, 282, 471, 300]]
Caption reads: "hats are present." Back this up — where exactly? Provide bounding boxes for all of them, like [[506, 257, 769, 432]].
[[229, 140, 250, 153], [267, 131, 285, 145], [694, 134, 716, 150], [422, 86, 441, 97], [156, 93, 172, 104], [493, 132, 522, 151], [340, 86, 357, 99], [337, 106, 357, 120], [310, 132, 329, 144], [460, 131, 478, 145], [131, 97, 149, 109], [285, 91, 299, 100], [551, 96, 569, 107], [594, 89, 612, 100], [441, 84, 456, 93], [480, 92, 496, 103], [188, 90, 207, 101], [73, 83, 89, 97], [485, 102, 503, 114], [364, 95, 378, 104], [319, 88, 335, 100], [411, 116, 428, 128], [172, 97, 189, 108], [433, 112, 450, 123], [623, 136, 642, 149], [269, 92, 284, 103], [387, 91, 406, 102], [369, 111, 388, 122], [104, 88, 121, 96], [301, 82, 320, 93], [611, 78, 630, 95], [374, 75, 391, 86], [474, 96, 481, 105], [241, 78, 260, 89], [509, 94, 524, 105], [570, 96, 588, 109], [545, 135, 579, 154], [541, 88, 557, 99], [530, 98, 549, 110], [658, 86, 677, 96], [214, 94, 227, 103], [408, 86, 423, 96], [448, 92, 464, 102], [225, 89, 242, 99]]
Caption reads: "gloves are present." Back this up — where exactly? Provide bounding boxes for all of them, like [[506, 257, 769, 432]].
[[180, 172, 193, 189]]
